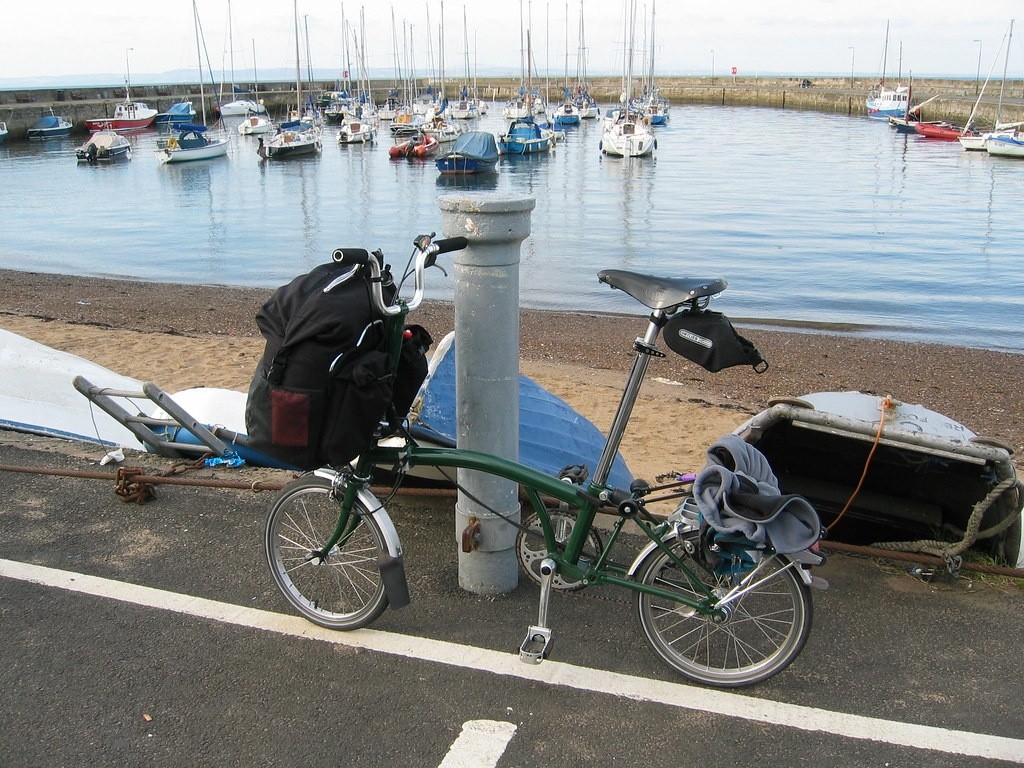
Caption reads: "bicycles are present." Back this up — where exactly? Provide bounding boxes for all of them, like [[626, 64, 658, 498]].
[[264, 236, 814, 690]]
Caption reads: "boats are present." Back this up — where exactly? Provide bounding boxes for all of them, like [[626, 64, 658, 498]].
[[0, 325, 635, 509], [0, 123, 9, 143], [26, 115, 73, 143], [433, 132, 500, 174], [154, 102, 196, 126], [727, 386, 1023, 569], [258, 127, 323, 159], [389, 131, 438, 156], [916, 121, 975, 141], [76, 129, 132, 160], [85, 100, 158, 133]]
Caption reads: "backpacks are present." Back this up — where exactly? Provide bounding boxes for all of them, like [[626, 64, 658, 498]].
[[245, 248, 398, 471]]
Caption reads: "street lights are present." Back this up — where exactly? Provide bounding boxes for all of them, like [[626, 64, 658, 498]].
[[711, 50, 714, 78], [126, 47, 134, 97], [972, 39, 981, 96], [849, 47, 855, 79]]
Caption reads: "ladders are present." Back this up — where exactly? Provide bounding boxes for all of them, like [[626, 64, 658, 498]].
[[72, 374, 235, 462]]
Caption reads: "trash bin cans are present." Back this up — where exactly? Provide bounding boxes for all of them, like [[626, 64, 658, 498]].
[[58, 90, 65, 101]]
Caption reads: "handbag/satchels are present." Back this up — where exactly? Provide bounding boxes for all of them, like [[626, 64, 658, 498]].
[[319, 320, 434, 467]]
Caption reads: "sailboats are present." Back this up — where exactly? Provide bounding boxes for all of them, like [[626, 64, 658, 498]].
[[865, 19, 949, 133], [958, 18, 1024, 157], [154, 1, 669, 162]]
